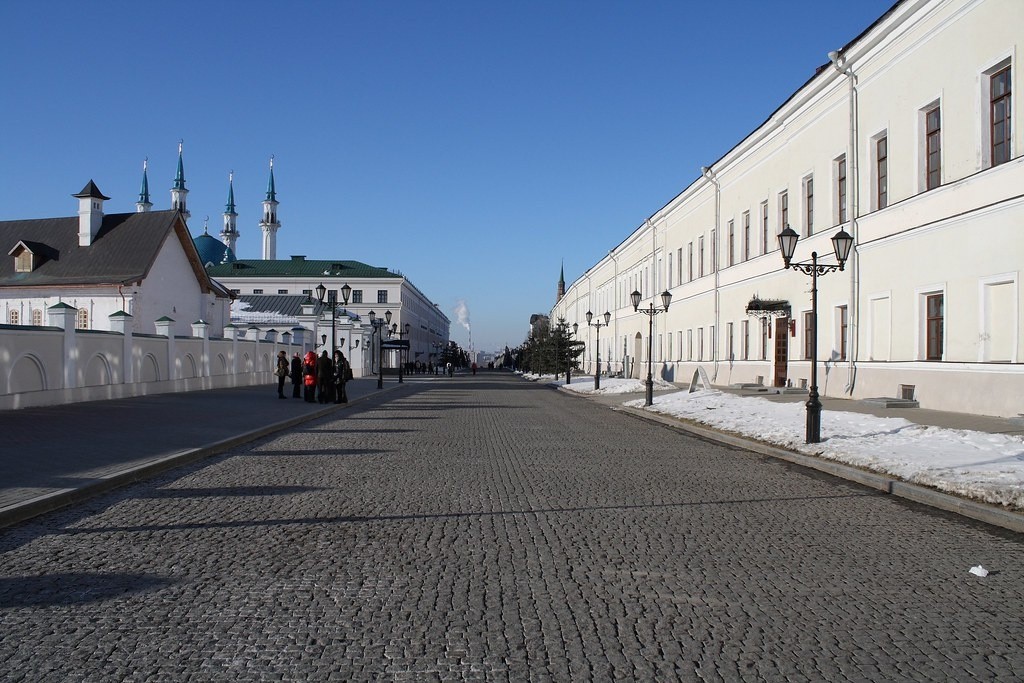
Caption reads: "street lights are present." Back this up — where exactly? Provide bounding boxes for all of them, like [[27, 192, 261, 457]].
[[368, 308, 393, 390], [315, 281, 352, 365], [586, 309, 611, 389], [630, 289, 673, 408], [431, 339, 444, 375], [776, 224, 855, 441], [393, 322, 411, 384], [558, 322, 579, 384]]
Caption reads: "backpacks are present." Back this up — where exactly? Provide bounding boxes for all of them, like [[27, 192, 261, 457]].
[[303, 359, 313, 375]]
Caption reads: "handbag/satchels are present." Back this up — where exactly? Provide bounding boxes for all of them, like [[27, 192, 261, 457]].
[[274, 368, 285, 378], [345, 368, 354, 382]]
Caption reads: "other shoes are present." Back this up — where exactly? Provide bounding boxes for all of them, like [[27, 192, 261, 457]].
[[288, 371, 292, 378], [342, 399, 348, 403], [279, 396, 288, 399], [334, 400, 342, 404], [309, 400, 317, 403]]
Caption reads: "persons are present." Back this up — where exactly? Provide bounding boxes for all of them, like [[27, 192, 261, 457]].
[[404, 360, 503, 378], [277, 350, 351, 405]]
